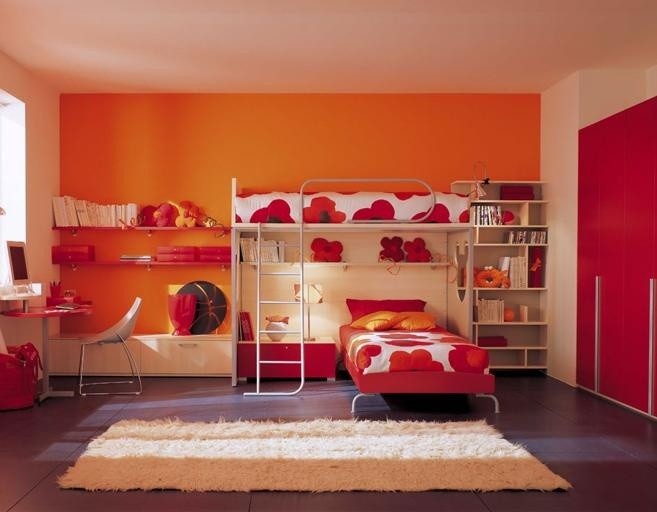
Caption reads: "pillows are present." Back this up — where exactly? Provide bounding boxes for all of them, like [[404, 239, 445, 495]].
[[347, 309, 411, 331], [344, 296, 430, 327], [389, 310, 440, 331]]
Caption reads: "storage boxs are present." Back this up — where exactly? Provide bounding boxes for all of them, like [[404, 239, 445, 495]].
[[472, 294, 505, 327]]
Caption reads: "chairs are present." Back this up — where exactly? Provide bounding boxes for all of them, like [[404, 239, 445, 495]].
[[72, 296, 144, 398]]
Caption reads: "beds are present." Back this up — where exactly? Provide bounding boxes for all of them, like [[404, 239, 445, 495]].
[[228, 174, 480, 385], [336, 318, 502, 421]]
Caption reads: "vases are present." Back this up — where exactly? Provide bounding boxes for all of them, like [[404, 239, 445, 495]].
[[264, 320, 290, 343]]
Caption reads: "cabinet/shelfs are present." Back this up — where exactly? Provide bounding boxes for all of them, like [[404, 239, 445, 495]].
[[45, 328, 234, 381], [447, 175, 550, 374], [50, 221, 234, 270]]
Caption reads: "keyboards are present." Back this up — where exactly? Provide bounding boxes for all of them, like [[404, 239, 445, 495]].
[[56, 303, 80, 309]]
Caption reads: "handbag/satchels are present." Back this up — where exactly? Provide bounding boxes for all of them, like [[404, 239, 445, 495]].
[[0, 342, 44, 411]]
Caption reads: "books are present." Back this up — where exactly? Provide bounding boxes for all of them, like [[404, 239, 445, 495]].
[[469, 204, 504, 225], [53, 195, 142, 228], [155, 244, 232, 262], [474, 295, 505, 322], [497, 254, 528, 288], [118, 254, 152, 261], [502, 229, 547, 244], [238, 311, 255, 341], [477, 335, 507, 348]]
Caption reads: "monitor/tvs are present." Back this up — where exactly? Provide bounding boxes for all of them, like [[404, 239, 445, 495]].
[[6, 241, 32, 285]]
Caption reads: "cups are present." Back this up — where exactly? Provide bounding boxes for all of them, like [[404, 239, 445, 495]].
[[519, 304, 528, 322], [49, 286, 60, 299], [169, 294, 197, 335]]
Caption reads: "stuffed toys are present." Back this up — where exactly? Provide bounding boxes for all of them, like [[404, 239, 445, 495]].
[[463, 264, 511, 289], [138, 199, 207, 227]]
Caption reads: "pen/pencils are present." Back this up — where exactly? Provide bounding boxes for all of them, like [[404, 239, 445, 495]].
[[50, 280, 63, 286]]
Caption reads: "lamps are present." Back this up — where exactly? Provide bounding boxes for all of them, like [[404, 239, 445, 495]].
[[293, 281, 326, 342]]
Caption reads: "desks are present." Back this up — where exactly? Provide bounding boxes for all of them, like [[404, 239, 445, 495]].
[[0, 286, 99, 408]]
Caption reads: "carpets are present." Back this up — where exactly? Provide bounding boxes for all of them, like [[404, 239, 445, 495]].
[[47, 411, 574, 494]]
